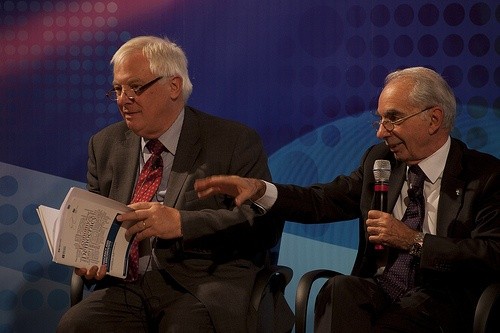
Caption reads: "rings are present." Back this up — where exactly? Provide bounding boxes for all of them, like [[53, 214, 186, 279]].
[[217, 186, 221, 192], [143, 220, 149, 228]]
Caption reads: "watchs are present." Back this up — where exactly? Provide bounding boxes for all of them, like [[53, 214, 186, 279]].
[[408, 231, 426, 259]]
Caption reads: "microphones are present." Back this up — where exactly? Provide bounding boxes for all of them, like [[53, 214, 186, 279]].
[[372, 160, 391, 250]]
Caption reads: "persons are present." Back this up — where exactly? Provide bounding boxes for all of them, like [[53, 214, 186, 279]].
[[56, 35, 275, 333], [193, 65, 500, 333]]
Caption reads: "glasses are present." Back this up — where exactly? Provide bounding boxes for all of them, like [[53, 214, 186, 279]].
[[372, 105, 437, 132], [105, 76, 163, 101]]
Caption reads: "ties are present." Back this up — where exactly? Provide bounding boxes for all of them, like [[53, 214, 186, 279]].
[[125, 139, 164, 285], [380, 166, 427, 301]]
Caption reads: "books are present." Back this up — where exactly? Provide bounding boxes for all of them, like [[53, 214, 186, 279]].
[[35, 186, 138, 280]]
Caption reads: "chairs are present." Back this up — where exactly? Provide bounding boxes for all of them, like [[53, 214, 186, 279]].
[[295, 268, 499, 333], [68, 264, 294, 333]]
